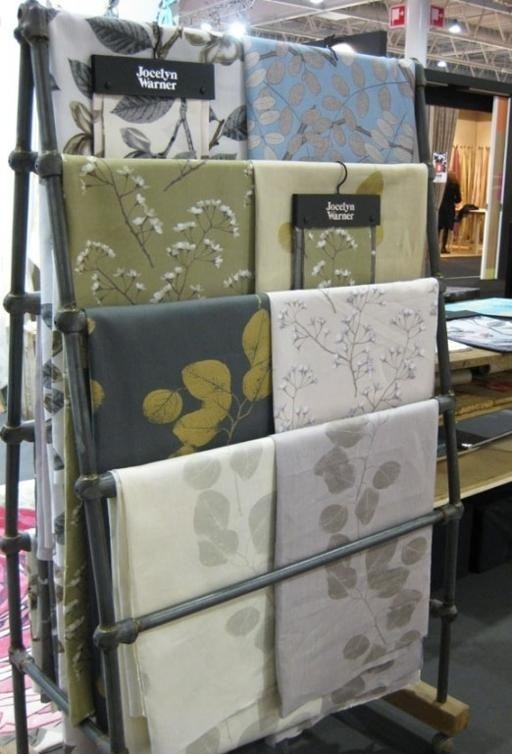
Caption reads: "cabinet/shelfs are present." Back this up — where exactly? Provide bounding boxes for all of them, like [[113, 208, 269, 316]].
[[434, 347, 512, 512]]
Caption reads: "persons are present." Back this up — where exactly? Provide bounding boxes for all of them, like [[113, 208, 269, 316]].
[[438, 172, 462, 254]]
